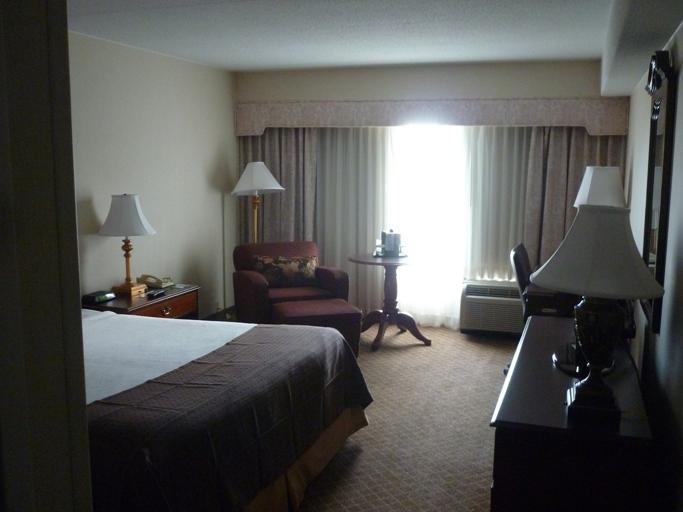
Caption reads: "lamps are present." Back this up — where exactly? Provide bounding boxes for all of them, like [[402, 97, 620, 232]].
[[529, 165, 664, 425], [97, 192, 156, 296], [229, 161, 285, 245]]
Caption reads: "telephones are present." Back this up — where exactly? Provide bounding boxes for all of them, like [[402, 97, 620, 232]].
[[136, 274, 175, 289]]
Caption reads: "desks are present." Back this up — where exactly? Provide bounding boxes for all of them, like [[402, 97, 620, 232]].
[[347, 252, 432, 350]]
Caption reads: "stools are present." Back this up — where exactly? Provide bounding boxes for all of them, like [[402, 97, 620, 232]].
[[271, 297, 362, 359]]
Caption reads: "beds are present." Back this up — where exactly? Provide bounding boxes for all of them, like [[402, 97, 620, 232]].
[[81, 309, 351, 511]]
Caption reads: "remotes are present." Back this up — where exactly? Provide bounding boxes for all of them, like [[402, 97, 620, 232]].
[[146, 289, 165, 298]]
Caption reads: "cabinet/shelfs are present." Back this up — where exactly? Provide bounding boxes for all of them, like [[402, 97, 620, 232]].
[[488, 314, 654, 511]]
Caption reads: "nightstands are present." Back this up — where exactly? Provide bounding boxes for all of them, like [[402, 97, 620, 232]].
[[80, 282, 201, 320]]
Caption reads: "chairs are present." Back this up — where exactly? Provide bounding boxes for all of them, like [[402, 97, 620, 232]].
[[507, 243, 577, 324], [231, 239, 349, 324]]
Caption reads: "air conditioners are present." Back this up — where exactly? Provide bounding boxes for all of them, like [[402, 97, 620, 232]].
[[457, 281, 525, 335]]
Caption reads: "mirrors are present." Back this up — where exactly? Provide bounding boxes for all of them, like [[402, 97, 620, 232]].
[[639, 50, 677, 336]]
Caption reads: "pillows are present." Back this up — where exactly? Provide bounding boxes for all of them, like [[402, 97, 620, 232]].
[[243, 252, 319, 288]]
